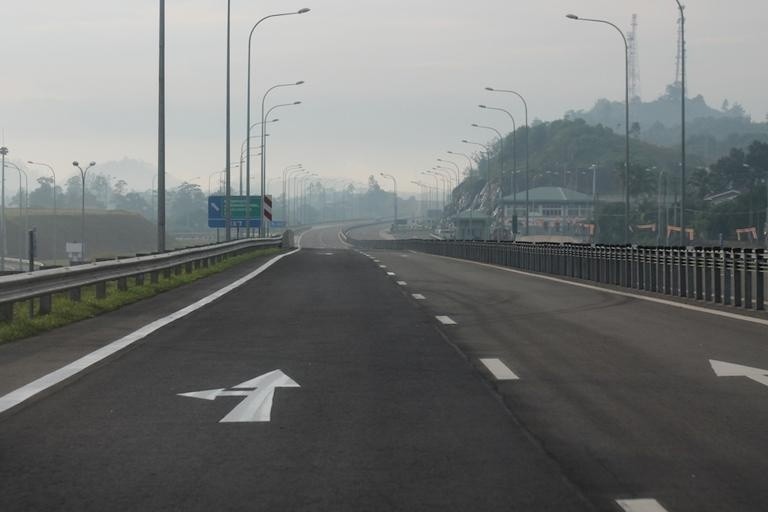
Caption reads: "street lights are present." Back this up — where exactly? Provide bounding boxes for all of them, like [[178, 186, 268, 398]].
[[0, 147, 30, 275], [72, 161, 95, 263], [27, 161, 56, 267], [207, 80, 318, 241], [247, 7, 311, 240], [565, 15, 631, 246], [377, 87, 529, 242]]
